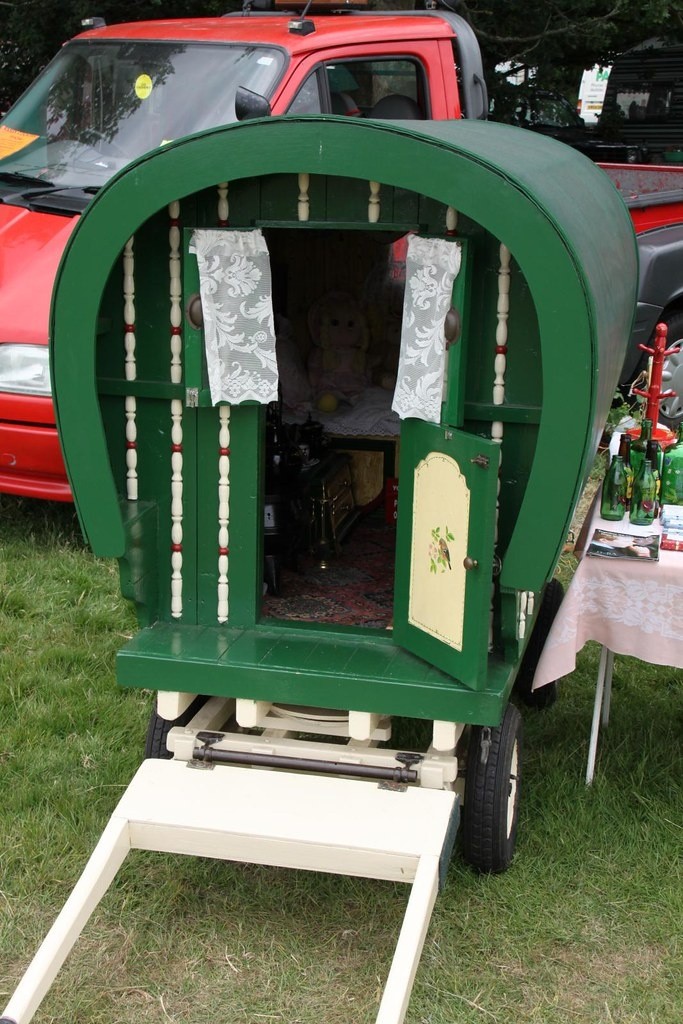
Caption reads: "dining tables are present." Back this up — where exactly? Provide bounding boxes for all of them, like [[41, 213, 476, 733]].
[[528, 479, 683, 792]]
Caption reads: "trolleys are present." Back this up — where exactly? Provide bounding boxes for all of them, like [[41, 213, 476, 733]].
[[1, 120, 641, 1024]]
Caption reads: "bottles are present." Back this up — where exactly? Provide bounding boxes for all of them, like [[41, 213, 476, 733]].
[[598, 414, 683, 526]]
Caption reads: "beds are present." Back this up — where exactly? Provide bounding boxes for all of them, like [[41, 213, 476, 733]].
[[270, 364, 403, 512]]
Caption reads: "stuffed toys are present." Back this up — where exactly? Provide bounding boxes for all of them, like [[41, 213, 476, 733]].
[[306, 285, 397, 408]]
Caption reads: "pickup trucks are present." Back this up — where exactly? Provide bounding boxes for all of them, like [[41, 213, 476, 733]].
[[0, 10, 682, 504]]
